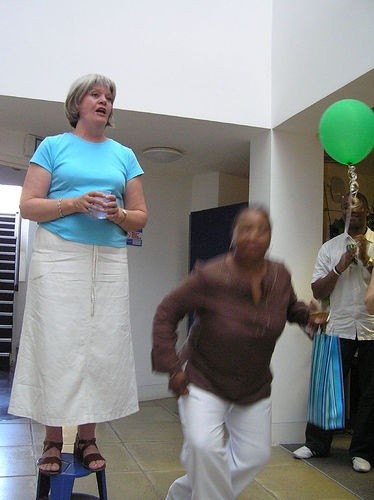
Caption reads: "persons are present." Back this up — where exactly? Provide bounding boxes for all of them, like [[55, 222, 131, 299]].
[[150, 209, 322, 500], [292, 193, 374, 471], [7, 74, 148, 474], [365, 257, 374, 315]]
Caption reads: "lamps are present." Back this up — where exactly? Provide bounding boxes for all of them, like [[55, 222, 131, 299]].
[[142, 147, 184, 163]]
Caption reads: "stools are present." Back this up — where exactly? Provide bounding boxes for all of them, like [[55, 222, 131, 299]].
[[36, 453, 108, 500]]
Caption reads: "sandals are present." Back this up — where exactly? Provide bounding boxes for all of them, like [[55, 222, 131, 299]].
[[72, 434, 106, 471], [39, 440, 63, 475]]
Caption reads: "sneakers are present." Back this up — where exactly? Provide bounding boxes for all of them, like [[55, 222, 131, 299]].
[[293, 447, 320, 458], [353, 456, 372, 471]]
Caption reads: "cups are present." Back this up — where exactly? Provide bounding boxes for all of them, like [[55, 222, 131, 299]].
[[93, 190, 111, 219]]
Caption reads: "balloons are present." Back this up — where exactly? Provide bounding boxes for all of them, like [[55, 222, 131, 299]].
[[319, 99, 374, 166]]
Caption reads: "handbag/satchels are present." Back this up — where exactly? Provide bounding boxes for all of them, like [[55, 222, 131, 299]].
[[306, 321, 344, 429]]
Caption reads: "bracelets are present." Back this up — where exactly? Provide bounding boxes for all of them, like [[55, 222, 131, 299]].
[[119, 209, 127, 224], [58, 199, 64, 217], [366, 258, 374, 272], [333, 266, 342, 275]]
